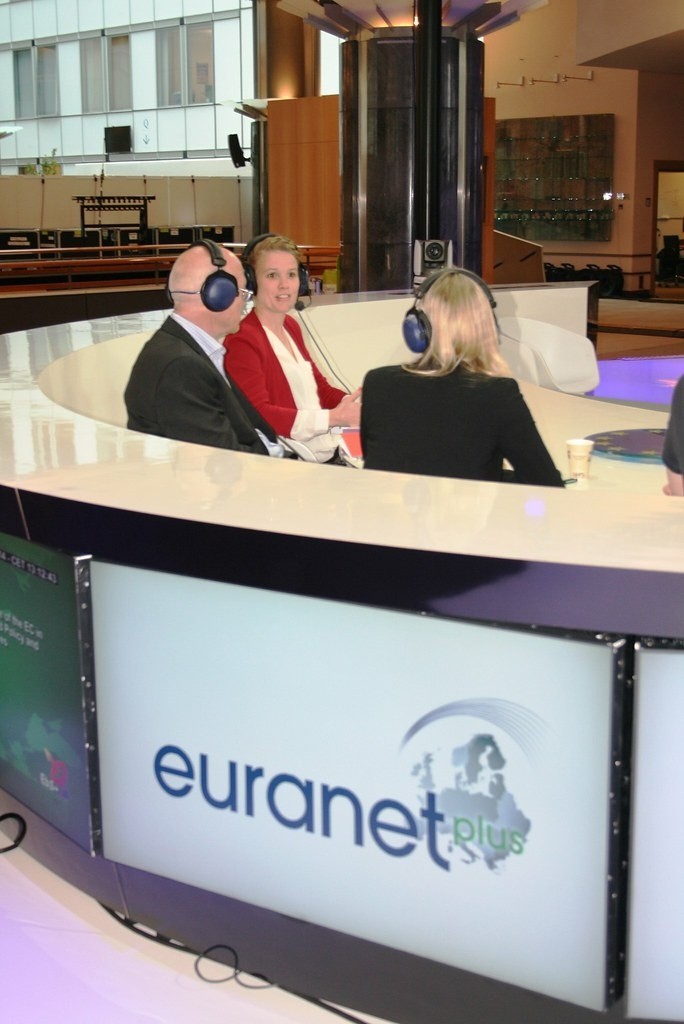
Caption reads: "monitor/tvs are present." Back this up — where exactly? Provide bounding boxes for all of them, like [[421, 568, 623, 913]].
[[105, 126, 131, 153]]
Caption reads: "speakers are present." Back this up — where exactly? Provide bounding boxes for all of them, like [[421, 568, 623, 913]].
[[228, 134, 246, 168]]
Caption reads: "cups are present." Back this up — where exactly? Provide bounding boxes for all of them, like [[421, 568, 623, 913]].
[[567, 439, 593, 480]]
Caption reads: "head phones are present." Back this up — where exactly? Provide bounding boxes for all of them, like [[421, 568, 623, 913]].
[[239, 233, 309, 296], [167, 239, 239, 312], [402, 268, 500, 353]]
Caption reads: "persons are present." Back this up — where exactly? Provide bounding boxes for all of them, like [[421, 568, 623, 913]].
[[223, 236, 364, 468], [661, 373, 684, 496], [360, 267, 566, 488], [124, 244, 299, 467]]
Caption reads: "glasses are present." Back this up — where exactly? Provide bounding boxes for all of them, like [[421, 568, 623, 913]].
[[239, 288, 253, 301]]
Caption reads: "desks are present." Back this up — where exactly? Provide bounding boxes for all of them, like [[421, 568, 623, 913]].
[[502, 378, 671, 489]]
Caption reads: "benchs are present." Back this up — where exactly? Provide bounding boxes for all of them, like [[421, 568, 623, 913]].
[[0, 280, 684, 574]]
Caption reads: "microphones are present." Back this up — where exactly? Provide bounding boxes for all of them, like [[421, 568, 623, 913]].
[[295, 290, 313, 311]]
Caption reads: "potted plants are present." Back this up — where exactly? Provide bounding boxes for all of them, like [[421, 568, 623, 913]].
[[18, 162, 38, 175], [39, 148, 65, 176]]
